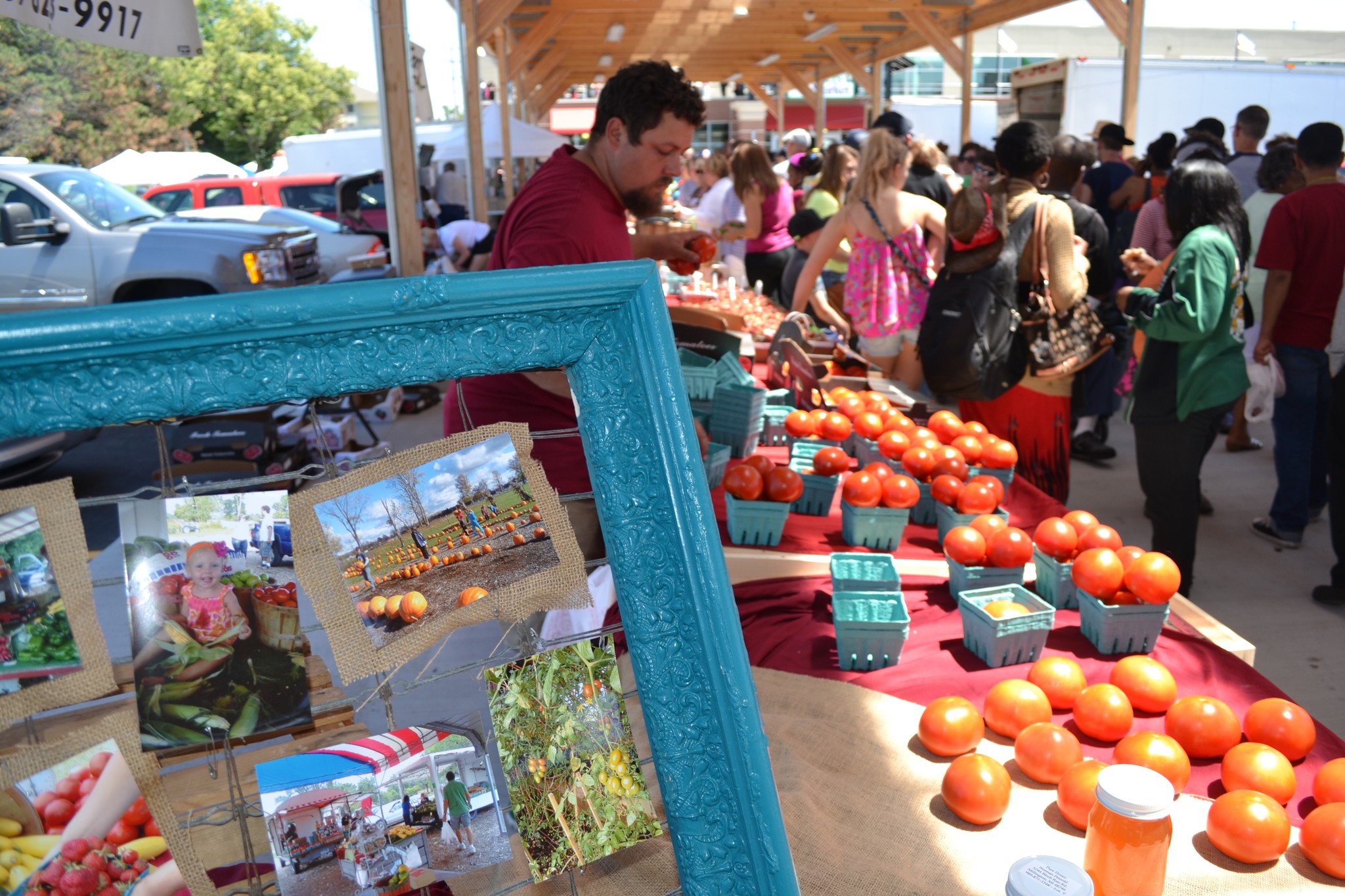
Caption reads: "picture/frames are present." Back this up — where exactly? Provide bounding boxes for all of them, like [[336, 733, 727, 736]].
[[0, 259, 797, 896]]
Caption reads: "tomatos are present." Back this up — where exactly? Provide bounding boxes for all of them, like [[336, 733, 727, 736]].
[[148, 574, 192, 595], [943, 510, 1181, 618], [528, 680, 639, 798], [34, 752, 161, 846], [724, 386, 1018, 515], [680, 283, 868, 377], [282, 842, 320, 853], [220, 569, 297, 608], [667, 259, 695, 276], [919, 654, 1345, 880], [690, 236, 715, 264]]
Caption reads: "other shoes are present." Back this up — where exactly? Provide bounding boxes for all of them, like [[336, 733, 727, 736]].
[[1225, 436, 1263, 452], [1070, 431, 1116, 460], [1312, 585, 1345, 606], [257, 564, 265, 568], [262, 565, 271, 570], [1198, 493, 1212, 515]]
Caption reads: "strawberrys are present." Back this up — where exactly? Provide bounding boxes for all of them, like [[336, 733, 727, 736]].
[[25, 836, 149, 896]]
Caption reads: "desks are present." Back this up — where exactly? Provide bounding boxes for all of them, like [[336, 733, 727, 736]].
[[654, 257, 1345, 896]]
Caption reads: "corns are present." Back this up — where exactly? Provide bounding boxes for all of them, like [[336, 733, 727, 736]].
[[145, 613, 261, 745]]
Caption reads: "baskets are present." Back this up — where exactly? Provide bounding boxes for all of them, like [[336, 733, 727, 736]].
[[672, 347, 1168, 669]]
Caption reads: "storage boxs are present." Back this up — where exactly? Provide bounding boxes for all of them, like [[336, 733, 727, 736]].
[[666, 306, 1169, 671]]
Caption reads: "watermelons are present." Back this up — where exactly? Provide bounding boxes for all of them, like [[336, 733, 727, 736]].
[[125, 535, 190, 575]]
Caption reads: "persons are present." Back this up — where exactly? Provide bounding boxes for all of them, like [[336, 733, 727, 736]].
[[401, 795, 414, 828], [456, 505, 499, 536], [257, 505, 275, 570], [663, 105, 1345, 616], [231, 513, 252, 560], [421, 219, 495, 272], [443, 60, 719, 557], [420, 793, 429, 804], [411, 526, 430, 561], [435, 163, 468, 226], [442, 771, 477, 854], [286, 811, 366, 843], [133, 540, 252, 687], [40, 747, 187, 896], [352, 547, 376, 591], [40, 544, 56, 584]]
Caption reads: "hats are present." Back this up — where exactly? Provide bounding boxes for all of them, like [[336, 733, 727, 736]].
[[789, 152, 806, 167], [1184, 119, 1224, 143], [871, 112, 910, 134], [1093, 124, 1135, 145], [843, 128, 870, 149], [946, 183, 1007, 272], [782, 128, 812, 147], [787, 209, 831, 240]]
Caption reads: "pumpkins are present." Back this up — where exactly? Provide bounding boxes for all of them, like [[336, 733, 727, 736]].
[[343, 501, 546, 622], [388, 864, 409, 887]]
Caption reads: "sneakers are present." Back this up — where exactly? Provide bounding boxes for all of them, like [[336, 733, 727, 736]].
[[467, 847, 477, 856], [1250, 518, 1301, 549], [456, 843, 466, 850]]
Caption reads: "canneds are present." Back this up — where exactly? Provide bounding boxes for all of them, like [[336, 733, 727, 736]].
[[316, 816, 342, 838], [1085, 763, 1175, 896]]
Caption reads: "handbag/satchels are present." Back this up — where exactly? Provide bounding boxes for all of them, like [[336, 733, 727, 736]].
[[441, 821, 458, 846], [1245, 353, 1277, 423], [1023, 194, 1115, 377]]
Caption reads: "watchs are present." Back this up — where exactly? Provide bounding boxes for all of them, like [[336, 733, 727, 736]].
[[443, 813, 446, 815]]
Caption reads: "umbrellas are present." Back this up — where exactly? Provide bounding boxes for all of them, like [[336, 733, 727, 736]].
[[429, 99, 573, 220]]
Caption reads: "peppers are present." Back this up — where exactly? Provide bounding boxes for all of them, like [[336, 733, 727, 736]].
[[19, 610, 79, 665]]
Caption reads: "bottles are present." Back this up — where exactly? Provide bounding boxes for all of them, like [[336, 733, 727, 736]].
[[1006, 855, 1094, 896], [315, 821, 320, 830], [1085, 764, 1175, 896]]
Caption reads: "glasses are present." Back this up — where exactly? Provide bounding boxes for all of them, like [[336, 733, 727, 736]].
[[696, 170, 704, 173], [959, 155, 978, 164], [974, 166, 995, 176]]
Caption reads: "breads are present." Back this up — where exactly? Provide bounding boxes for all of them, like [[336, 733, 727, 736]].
[[339, 823, 386, 854]]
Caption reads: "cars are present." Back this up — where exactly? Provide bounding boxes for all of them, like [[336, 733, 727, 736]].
[[0, 159, 434, 311]]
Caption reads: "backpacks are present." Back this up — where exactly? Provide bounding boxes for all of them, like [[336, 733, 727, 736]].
[[917, 199, 1032, 407]]
